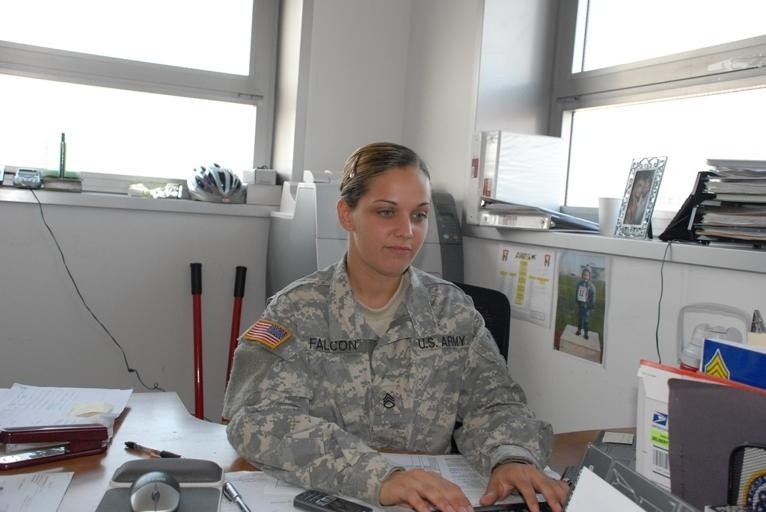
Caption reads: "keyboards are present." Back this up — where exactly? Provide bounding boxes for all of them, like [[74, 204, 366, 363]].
[[426, 501, 562, 512]]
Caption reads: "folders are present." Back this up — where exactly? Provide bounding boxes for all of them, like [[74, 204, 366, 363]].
[[478, 195, 601, 232]]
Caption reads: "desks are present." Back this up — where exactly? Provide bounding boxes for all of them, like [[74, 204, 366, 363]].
[[0, 387, 641, 510]]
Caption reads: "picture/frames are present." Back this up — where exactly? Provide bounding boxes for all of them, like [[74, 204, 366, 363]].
[[612, 155, 667, 238]]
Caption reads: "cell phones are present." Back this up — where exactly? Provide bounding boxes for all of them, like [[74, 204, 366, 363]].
[[294, 488, 373, 511]]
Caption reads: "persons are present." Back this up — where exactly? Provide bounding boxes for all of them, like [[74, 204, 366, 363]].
[[220, 142, 572, 511], [624, 178, 644, 224], [634, 176, 652, 224], [576, 265, 596, 339]]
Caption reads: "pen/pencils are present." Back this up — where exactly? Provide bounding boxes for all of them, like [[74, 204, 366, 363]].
[[125, 441, 183, 458], [224, 482, 251, 512]]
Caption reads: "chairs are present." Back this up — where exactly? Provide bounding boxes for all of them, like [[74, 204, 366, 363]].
[[437, 279, 510, 454]]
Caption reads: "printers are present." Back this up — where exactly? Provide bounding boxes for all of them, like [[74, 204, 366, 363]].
[[264, 167, 464, 306]]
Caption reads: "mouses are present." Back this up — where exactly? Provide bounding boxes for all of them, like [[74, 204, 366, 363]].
[[129, 469, 180, 512]]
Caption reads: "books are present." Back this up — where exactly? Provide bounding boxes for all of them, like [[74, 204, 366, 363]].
[[697, 159, 766, 250]]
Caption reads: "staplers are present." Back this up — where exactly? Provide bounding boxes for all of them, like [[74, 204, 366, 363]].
[[0, 424, 106, 471]]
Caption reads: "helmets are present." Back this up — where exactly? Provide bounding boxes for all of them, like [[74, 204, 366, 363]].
[[187, 162, 245, 203]]
